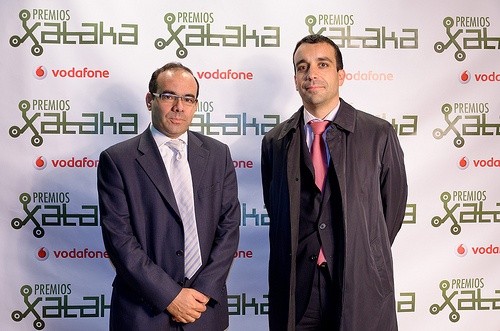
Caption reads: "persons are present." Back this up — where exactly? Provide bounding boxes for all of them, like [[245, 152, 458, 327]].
[[261, 35, 408, 331], [97, 62, 241, 331]]
